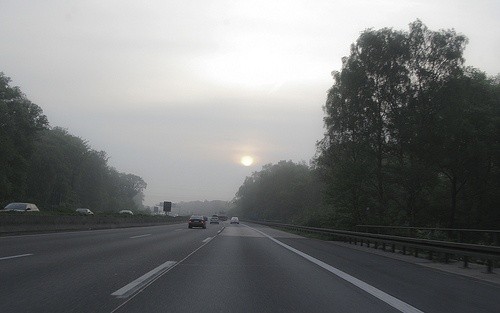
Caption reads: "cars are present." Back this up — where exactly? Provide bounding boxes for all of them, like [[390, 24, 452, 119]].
[[230, 216, 239, 224], [0, 202, 40, 213], [188, 215, 206, 229], [76, 208, 94, 216], [120, 209, 134, 216], [210, 217, 220, 224]]
